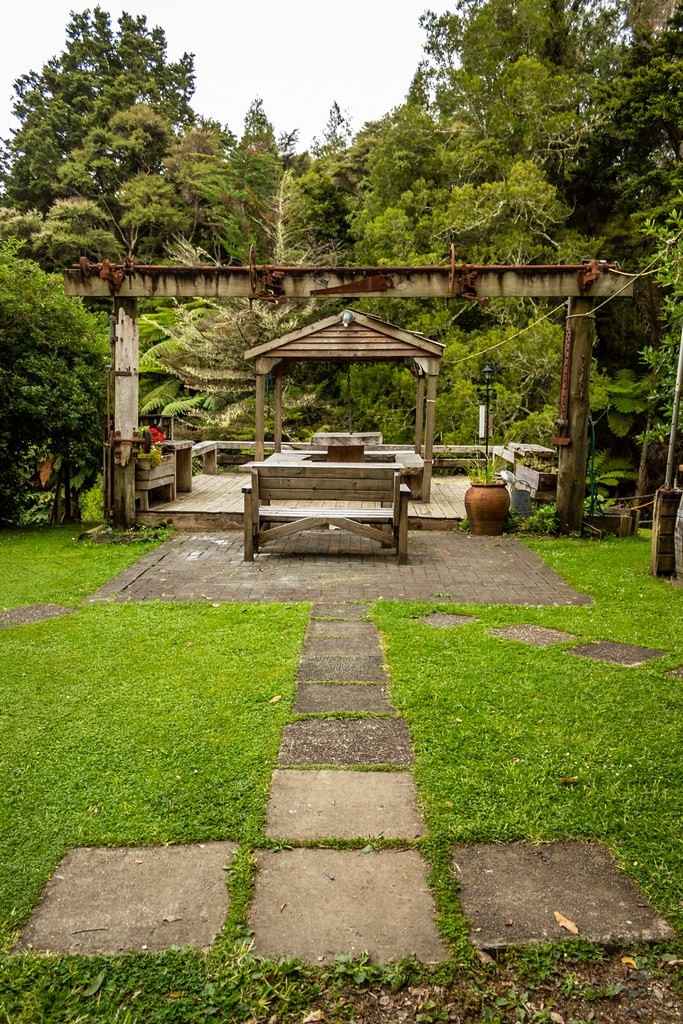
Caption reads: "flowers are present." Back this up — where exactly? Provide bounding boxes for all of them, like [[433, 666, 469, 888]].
[[138, 426, 167, 467]]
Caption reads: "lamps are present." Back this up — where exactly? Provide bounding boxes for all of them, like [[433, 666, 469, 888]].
[[340, 310, 354, 328], [482, 365, 494, 381]]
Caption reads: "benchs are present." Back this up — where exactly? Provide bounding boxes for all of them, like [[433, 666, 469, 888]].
[[264, 449, 424, 500], [242, 466, 411, 566]]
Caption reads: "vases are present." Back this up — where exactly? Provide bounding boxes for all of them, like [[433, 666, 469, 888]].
[[135, 456, 176, 490]]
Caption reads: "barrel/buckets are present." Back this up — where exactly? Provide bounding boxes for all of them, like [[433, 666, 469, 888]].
[[510, 479, 532, 513]]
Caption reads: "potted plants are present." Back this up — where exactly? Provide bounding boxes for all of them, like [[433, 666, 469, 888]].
[[452, 448, 511, 537], [515, 454, 559, 491]]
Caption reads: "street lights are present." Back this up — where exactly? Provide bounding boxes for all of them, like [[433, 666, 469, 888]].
[[480, 364, 495, 475]]
